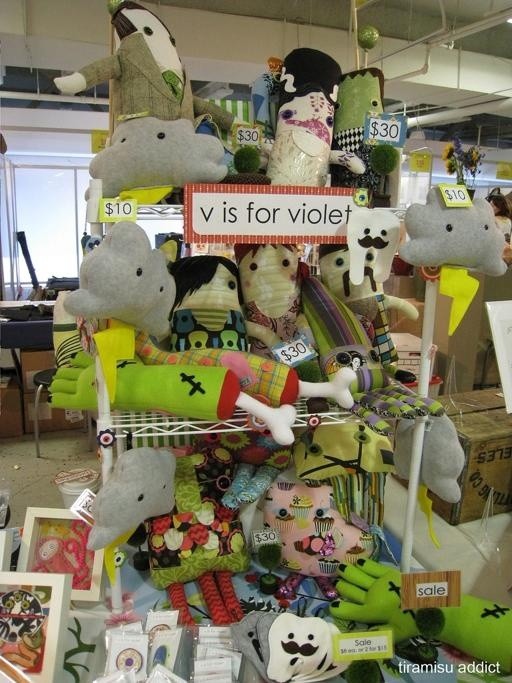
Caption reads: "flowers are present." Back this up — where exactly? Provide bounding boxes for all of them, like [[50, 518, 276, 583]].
[[442, 136, 486, 188]]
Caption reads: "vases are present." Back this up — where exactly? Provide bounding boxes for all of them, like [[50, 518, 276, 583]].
[[467, 189, 476, 201]]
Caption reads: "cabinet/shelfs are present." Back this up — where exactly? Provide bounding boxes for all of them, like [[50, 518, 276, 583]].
[[86, 178, 439, 615]]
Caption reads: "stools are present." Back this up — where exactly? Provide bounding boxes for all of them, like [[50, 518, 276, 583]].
[[30, 369, 93, 458]]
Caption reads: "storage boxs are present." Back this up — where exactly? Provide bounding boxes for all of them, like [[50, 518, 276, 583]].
[[21, 349, 88, 392], [383, 275, 415, 298], [22, 394, 87, 434], [0, 389, 23, 440], [403, 375, 443, 396], [387, 332, 439, 383], [386, 298, 424, 337], [391, 388, 512, 526]]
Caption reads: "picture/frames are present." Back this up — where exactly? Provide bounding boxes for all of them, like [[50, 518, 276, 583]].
[[0, 572, 74, 682], [15, 506, 104, 602]]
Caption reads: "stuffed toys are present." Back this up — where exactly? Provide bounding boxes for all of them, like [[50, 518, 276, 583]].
[[53, 0, 512, 682]]
